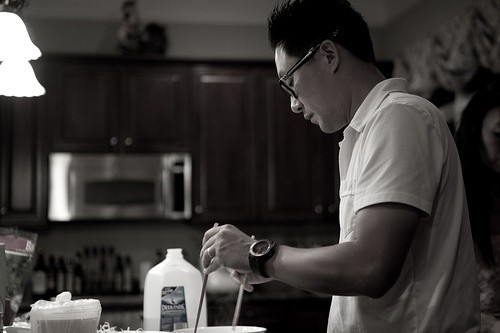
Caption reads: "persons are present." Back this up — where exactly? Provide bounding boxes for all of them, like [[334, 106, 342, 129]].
[[451, 72, 500, 266], [201, 0, 482, 333]]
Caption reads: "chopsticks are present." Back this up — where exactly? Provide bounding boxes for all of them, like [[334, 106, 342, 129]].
[[194, 223, 255, 333]]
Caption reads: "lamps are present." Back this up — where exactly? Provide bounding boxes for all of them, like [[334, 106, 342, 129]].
[[0, 0, 46, 98]]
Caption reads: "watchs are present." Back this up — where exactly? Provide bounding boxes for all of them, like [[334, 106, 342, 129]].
[[249, 238, 280, 278]]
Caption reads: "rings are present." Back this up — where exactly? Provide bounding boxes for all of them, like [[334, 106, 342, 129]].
[[208, 247, 214, 259]]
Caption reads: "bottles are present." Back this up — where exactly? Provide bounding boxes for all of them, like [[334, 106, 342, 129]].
[[29, 248, 133, 300], [143, 249, 207, 331]]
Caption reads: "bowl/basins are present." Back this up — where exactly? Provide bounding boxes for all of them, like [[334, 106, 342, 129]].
[[174, 326, 267, 333]]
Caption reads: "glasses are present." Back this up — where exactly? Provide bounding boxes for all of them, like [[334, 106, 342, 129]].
[[278, 41, 320, 98]]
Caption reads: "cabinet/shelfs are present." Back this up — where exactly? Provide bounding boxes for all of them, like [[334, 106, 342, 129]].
[[2, 52, 394, 237]]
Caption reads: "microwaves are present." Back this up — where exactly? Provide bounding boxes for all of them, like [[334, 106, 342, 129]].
[[48, 152, 192, 221]]
